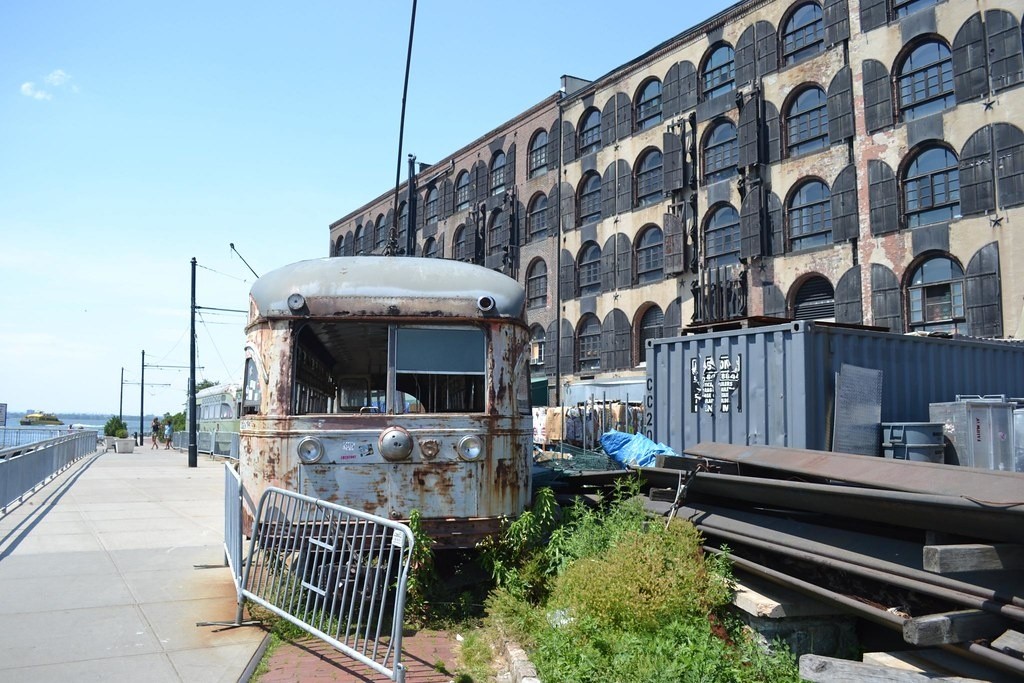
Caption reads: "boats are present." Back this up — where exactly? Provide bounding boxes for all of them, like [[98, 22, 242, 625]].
[[19, 410, 66, 426]]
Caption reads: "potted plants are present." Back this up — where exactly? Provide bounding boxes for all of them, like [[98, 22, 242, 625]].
[[103, 415, 137, 453]]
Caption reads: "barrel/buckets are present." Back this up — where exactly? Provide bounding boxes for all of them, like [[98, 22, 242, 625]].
[[882, 422, 945, 465]]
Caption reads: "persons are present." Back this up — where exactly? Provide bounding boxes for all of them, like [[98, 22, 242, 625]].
[[67, 425, 74, 433], [165, 419, 172, 450], [150, 417, 163, 449]]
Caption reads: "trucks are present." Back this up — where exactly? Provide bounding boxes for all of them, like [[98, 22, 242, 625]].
[[236, 252, 535, 574]]
